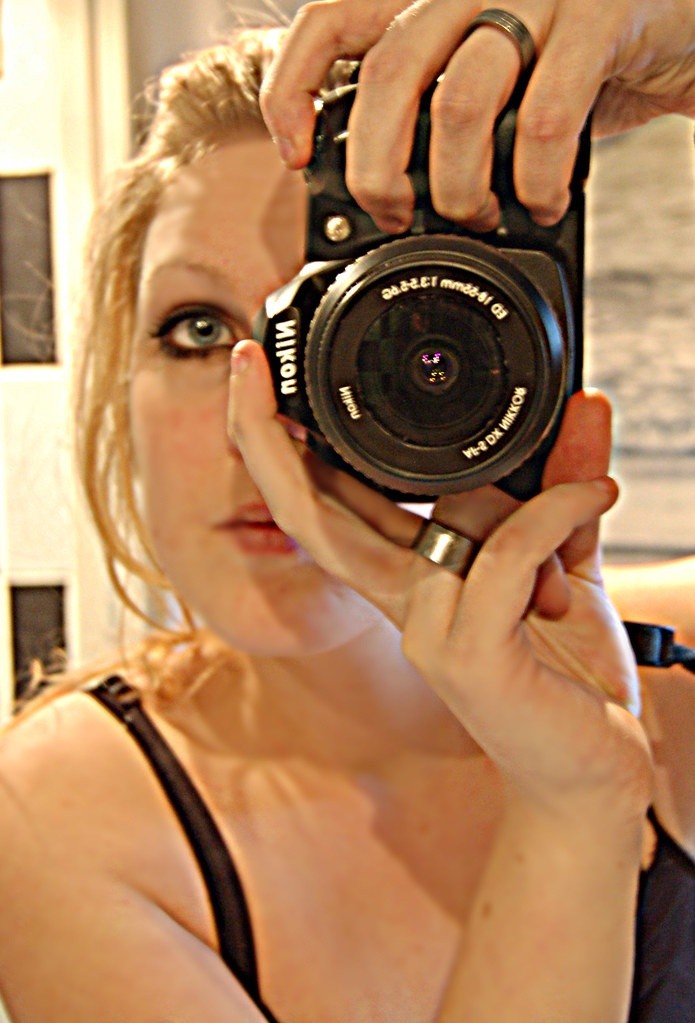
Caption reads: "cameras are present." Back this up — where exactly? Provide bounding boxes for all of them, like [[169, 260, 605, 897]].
[[260, 60, 590, 494]]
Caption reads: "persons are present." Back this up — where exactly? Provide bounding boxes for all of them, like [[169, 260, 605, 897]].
[[0, 0, 695, 1023]]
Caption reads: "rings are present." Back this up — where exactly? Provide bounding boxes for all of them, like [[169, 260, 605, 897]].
[[411, 520, 481, 578], [462, 7, 535, 71]]
